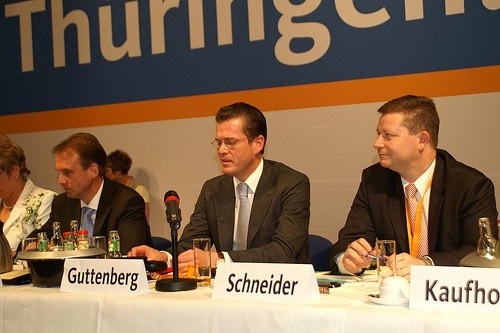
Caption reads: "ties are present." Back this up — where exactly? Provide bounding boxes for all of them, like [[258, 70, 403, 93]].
[[235, 183, 250, 251], [80, 208, 94, 244], [405, 183, 428, 259]]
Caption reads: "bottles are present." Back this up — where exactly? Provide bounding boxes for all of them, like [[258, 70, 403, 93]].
[[475, 216, 500, 258], [35, 220, 90, 252], [106, 230, 122, 259]]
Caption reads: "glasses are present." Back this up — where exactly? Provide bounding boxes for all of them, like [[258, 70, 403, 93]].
[[211, 134, 259, 149]]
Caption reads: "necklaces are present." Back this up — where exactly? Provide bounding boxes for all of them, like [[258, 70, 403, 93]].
[[2, 200, 14, 209]]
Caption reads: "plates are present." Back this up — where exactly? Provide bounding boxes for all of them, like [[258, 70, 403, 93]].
[[367, 291, 408, 306]]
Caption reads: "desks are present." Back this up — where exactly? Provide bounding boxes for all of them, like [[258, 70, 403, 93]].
[[0, 271, 500, 333]]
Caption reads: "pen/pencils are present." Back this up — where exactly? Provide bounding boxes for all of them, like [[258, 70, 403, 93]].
[[366, 254, 386, 261]]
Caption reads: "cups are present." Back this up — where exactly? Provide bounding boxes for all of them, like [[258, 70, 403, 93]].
[[193, 238, 212, 288], [379, 275, 409, 300], [21, 237, 37, 252], [375, 239, 396, 283], [89, 235, 107, 259]]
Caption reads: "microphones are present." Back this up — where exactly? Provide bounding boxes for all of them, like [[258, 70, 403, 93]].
[[164, 190, 182, 230]]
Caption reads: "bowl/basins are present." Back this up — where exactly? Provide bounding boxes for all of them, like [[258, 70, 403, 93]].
[[18, 247, 105, 287]]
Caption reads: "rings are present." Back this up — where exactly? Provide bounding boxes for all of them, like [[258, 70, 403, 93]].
[[401, 268, 404, 277], [345, 249, 348, 256]]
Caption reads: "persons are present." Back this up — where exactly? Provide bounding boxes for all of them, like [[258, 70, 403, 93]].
[[13, 133, 153, 264], [0, 134, 59, 257], [329, 94, 499, 282], [127, 102, 311, 277], [105, 149, 149, 222]]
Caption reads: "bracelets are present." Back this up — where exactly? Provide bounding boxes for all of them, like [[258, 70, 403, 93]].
[[334, 253, 342, 263]]
